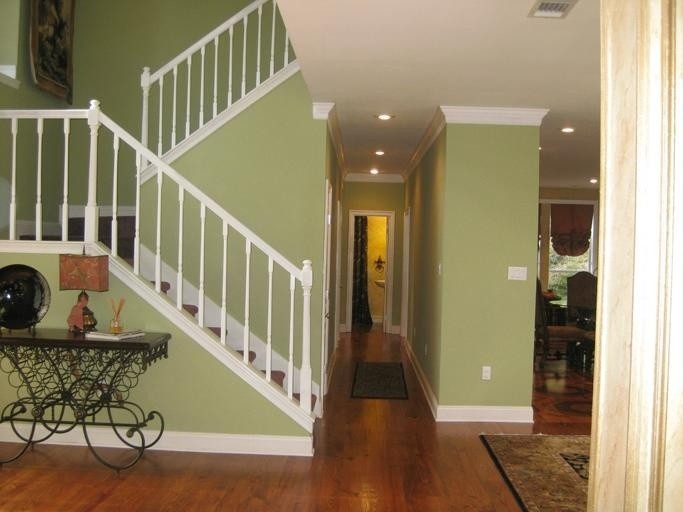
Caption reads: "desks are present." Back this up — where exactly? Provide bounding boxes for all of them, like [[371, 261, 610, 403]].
[[1, 324, 172, 474]]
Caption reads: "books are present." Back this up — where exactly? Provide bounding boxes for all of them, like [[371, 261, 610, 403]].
[[84, 328, 146, 341]]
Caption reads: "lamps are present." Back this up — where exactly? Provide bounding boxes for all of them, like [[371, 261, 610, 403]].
[[58, 253, 109, 330]]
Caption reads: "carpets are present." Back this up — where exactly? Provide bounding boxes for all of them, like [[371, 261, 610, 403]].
[[478, 424, 590, 511], [349, 358, 409, 400]]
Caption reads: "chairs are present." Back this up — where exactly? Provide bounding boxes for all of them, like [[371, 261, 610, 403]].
[[537, 269, 596, 369]]
